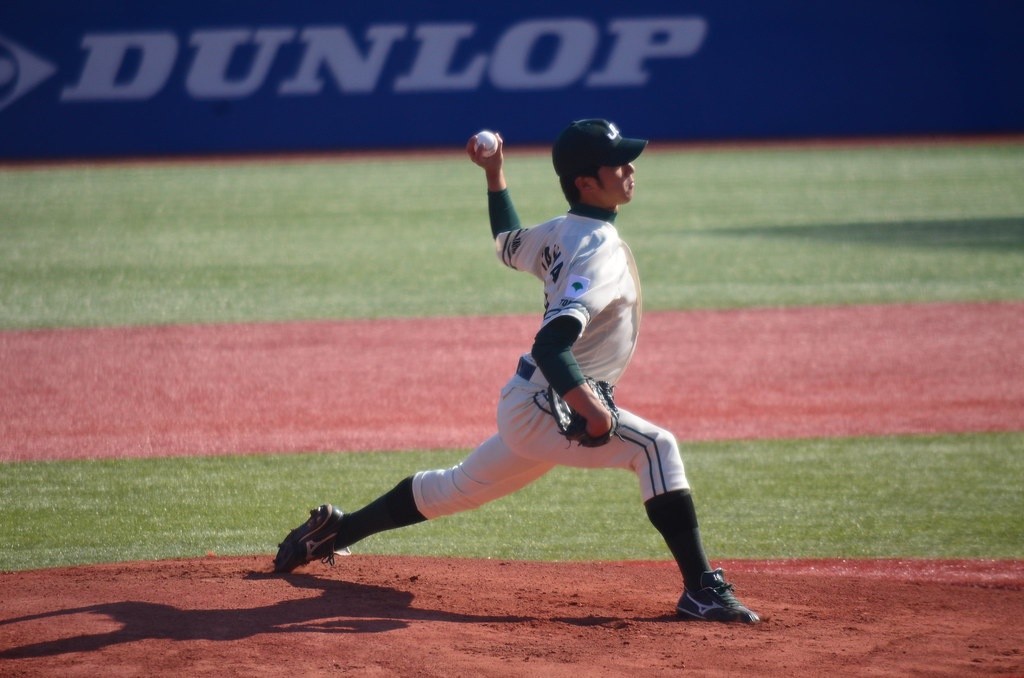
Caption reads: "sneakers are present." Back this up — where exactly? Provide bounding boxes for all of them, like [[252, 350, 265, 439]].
[[271, 505, 350, 572], [675, 567, 763, 623]]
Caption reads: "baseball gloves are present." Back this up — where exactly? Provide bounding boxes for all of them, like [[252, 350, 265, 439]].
[[548, 375, 620, 448]]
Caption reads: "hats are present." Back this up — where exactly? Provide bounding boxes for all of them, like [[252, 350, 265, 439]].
[[553, 119, 649, 177]]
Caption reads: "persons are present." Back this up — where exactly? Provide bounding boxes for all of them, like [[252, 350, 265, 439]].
[[272, 118, 759, 624]]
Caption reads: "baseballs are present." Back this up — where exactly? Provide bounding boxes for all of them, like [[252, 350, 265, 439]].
[[472, 130, 498, 158]]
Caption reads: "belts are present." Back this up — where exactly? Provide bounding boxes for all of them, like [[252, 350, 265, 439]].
[[515, 356, 536, 380]]
